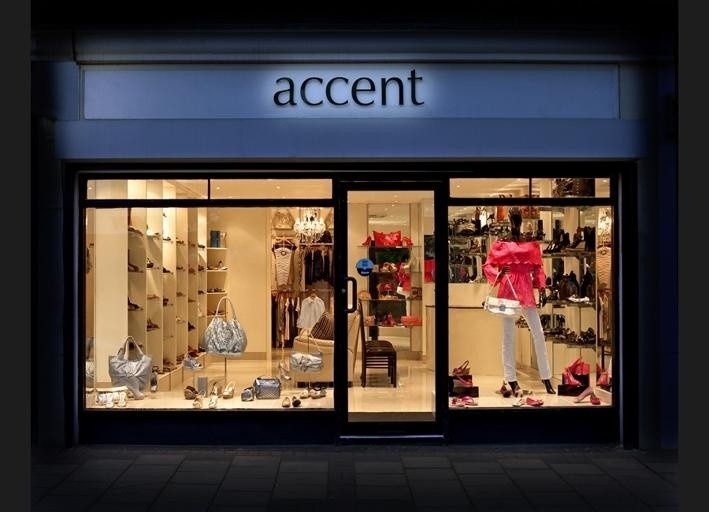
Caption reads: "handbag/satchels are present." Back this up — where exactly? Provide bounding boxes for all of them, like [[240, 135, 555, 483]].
[[364, 312, 420, 326], [241, 387, 254, 401], [569, 359, 590, 374], [109, 336, 151, 391], [373, 230, 400, 248], [204, 297, 247, 357], [540, 313, 563, 333], [484, 296, 521, 316], [96, 386, 128, 404], [289, 351, 322, 371], [256, 377, 279, 399]]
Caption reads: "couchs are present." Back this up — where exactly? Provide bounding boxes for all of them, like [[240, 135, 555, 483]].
[[292, 311, 360, 387]]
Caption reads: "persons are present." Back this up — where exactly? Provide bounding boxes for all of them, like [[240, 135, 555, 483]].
[[483, 207, 557, 398]]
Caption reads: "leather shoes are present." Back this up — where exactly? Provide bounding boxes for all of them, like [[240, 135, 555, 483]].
[[129, 386, 144, 400], [301, 389, 327, 399], [184, 354, 203, 368], [513, 399, 525, 406], [591, 394, 600, 404], [548, 327, 602, 343], [362, 237, 371, 245], [105, 393, 113, 408], [280, 360, 291, 380], [526, 398, 543, 405], [575, 386, 593, 402], [118, 392, 126, 407], [283, 396, 300, 407]]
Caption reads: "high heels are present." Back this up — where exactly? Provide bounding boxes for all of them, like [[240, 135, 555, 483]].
[[452, 360, 469, 376], [456, 376, 472, 387], [562, 368, 581, 385]]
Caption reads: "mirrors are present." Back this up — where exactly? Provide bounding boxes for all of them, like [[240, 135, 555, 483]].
[[595, 177, 615, 395], [366, 203, 412, 353]]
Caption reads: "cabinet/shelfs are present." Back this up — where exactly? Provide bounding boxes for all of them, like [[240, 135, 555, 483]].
[[448, 177, 598, 399], [357, 246, 423, 328], [92, 178, 229, 393]]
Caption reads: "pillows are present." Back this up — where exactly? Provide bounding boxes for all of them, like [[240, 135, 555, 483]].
[[310, 311, 333, 340]]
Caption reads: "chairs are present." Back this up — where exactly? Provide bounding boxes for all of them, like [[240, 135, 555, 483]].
[[358, 298, 398, 387]]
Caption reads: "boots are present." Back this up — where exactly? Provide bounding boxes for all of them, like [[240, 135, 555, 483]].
[[543, 227, 595, 253]]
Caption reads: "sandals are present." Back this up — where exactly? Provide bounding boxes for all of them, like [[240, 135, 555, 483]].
[[193, 381, 236, 408], [452, 396, 477, 406]]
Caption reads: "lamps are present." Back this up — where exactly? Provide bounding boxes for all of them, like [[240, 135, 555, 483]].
[[293, 206, 326, 242]]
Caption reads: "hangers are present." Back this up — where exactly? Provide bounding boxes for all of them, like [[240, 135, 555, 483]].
[[274, 235, 329, 255], [599, 237, 608, 254], [309, 290, 317, 299]]
